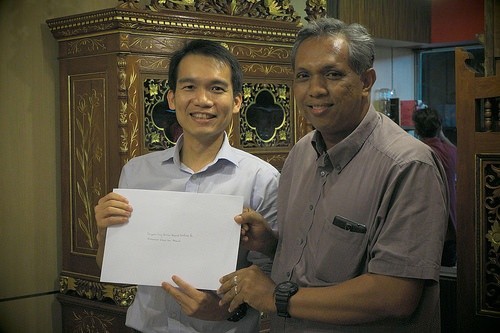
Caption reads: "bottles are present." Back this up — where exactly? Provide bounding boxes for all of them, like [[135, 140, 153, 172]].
[[380, 88, 391, 116]]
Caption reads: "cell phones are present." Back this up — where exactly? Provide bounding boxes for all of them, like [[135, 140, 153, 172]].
[[332, 215, 367, 234]]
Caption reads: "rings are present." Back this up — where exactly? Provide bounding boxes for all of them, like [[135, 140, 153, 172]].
[[235, 286, 238, 295], [234, 275, 239, 284]]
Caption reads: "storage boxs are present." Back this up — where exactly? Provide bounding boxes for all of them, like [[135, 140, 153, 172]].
[[401, 100, 423, 126]]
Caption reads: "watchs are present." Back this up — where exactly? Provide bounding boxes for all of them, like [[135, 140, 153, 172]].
[[273, 281, 299, 317], [227, 303, 248, 324]]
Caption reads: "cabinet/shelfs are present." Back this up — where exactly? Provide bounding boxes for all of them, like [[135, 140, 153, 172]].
[[455, 47, 500, 333], [45, 0, 327, 333]]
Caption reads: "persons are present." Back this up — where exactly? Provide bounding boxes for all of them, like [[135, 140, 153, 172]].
[[95, 40, 289, 333], [220, 18, 451, 333], [414, 108, 461, 267]]
